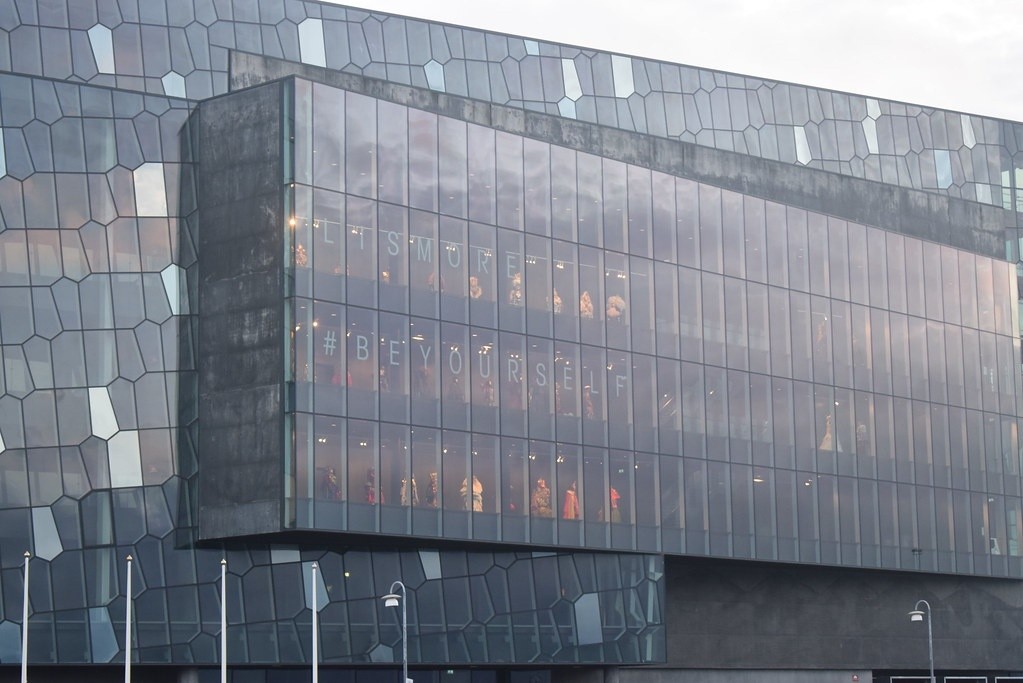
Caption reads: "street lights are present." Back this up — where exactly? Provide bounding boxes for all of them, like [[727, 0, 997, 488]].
[[908, 599, 936, 683], [380, 580, 408, 683]]
